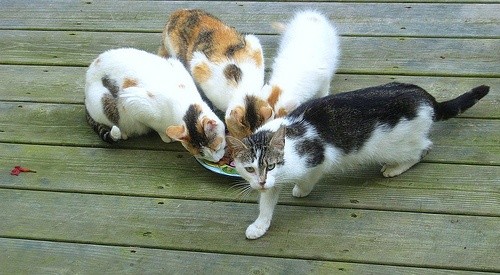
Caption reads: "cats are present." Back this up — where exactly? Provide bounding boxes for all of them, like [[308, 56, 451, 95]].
[[260, 8, 340, 119], [84, 48, 225, 162], [224, 83, 492, 239], [158, 8, 274, 138]]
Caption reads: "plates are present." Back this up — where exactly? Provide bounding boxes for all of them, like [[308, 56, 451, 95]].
[[194, 136, 252, 177]]
